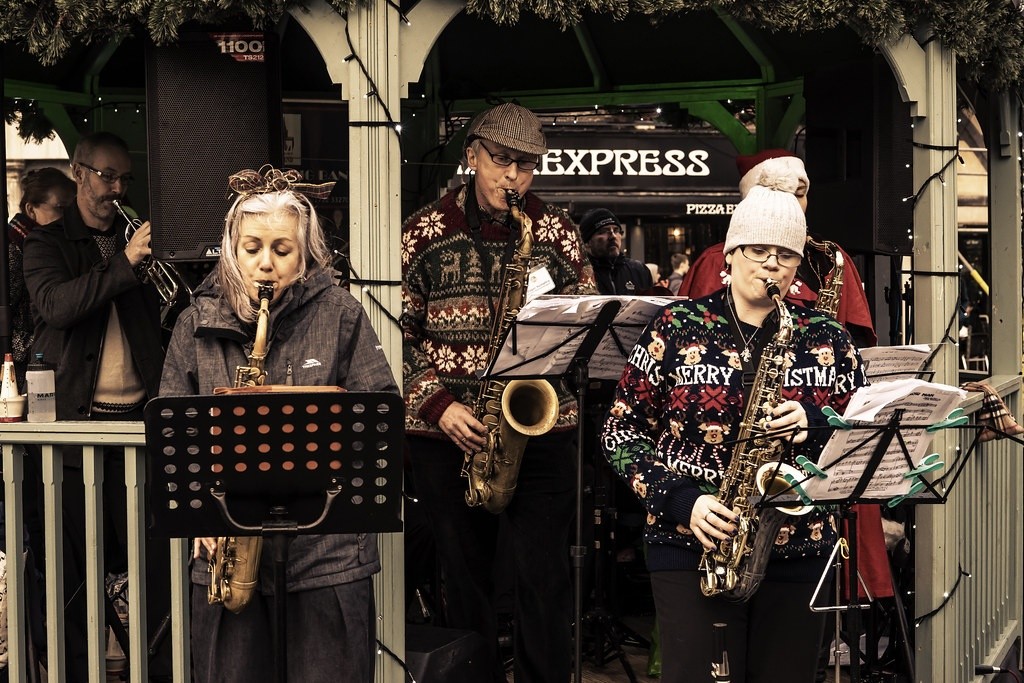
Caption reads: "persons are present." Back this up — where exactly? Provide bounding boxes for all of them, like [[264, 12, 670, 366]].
[[19, 130, 176, 682], [599, 187, 875, 683], [399, 99, 596, 682], [568, 205, 690, 672], [3, 165, 81, 392], [676, 146, 898, 682], [159, 161, 400, 683]]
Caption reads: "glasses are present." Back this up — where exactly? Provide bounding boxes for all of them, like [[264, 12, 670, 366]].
[[479, 141, 541, 171], [595, 229, 621, 235], [76, 162, 136, 185], [43, 201, 67, 213], [739, 245, 801, 268]]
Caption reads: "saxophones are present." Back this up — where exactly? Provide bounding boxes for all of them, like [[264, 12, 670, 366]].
[[805, 233, 847, 322], [457, 188, 563, 515], [205, 279, 278, 617], [696, 278, 818, 606]]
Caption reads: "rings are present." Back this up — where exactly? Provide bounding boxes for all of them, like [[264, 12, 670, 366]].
[[704, 511, 711, 521]]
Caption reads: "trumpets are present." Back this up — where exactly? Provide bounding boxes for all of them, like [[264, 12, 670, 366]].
[[110, 197, 196, 306]]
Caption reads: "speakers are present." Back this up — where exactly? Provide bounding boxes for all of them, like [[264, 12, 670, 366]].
[[803, 45, 914, 254], [140, 25, 284, 267]]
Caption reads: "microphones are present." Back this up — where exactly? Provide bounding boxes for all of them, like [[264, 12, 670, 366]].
[[975, 665, 1009, 675]]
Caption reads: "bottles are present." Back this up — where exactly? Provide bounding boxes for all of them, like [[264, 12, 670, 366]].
[[25, 353, 55, 422]]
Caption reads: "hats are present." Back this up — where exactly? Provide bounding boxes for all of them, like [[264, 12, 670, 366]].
[[579, 208, 624, 244], [736, 148, 810, 200], [463, 102, 548, 167], [723, 165, 807, 258]]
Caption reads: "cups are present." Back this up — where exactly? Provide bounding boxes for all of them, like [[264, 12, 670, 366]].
[[0, 396, 26, 423]]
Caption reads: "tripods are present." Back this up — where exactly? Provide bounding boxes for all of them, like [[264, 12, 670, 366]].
[[505, 379, 653, 683]]
